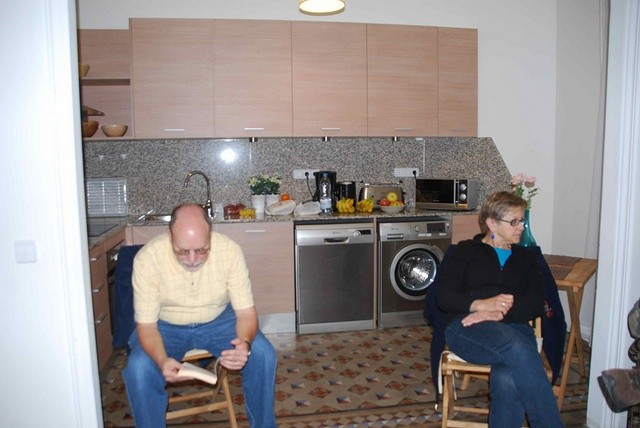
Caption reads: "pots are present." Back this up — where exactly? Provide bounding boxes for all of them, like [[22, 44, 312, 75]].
[[358, 179, 404, 209]]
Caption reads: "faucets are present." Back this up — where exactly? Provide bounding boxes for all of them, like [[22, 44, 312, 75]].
[[183, 169, 215, 218]]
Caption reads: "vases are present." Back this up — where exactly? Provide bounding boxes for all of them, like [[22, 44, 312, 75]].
[[251, 195, 265, 213], [514, 210, 538, 246], [266, 195, 279, 209], [508, 173, 540, 208]]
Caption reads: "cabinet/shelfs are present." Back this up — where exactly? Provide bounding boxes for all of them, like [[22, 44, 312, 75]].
[[75, 29, 132, 140], [293, 21, 367, 137], [437, 27, 478, 138], [89, 215, 138, 387], [214, 19, 292, 138], [367, 23, 438, 137], [129, 18, 215, 139]]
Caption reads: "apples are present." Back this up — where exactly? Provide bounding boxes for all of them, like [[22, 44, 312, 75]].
[[380, 198, 390, 206], [387, 191, 398, 202], [390, 201, 402, 207]]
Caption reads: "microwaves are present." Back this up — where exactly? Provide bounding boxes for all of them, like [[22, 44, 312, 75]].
[[415, 177, 478, 211]]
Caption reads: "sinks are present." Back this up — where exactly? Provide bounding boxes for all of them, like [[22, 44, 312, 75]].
[[136, 214, 171, 224]]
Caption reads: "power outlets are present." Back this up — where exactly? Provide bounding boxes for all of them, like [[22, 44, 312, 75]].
[[394, 167, 419, 177], [293, 170, 319, 180]]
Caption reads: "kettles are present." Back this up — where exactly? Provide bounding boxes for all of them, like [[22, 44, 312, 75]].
[[336, 181, 356, 213], [313, 171, 338, 214]]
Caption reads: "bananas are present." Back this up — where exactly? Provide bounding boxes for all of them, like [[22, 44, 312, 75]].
[[356, 195, 374, 212], [336, 197, 355, 213]]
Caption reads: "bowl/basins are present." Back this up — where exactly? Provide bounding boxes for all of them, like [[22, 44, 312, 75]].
[[101, 124, 128, 137], [380, 204, 407, 216], [78, 63, 90, 77], [81, 121, 99, 137]]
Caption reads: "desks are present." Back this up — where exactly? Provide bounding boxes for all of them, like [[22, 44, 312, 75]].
[[512, 254, 599, 412]]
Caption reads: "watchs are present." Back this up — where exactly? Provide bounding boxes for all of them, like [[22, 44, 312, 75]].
[[243, 341, 251, 362]]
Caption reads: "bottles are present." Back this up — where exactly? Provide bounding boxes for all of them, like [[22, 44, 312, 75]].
[[318, 172, 332, 215]]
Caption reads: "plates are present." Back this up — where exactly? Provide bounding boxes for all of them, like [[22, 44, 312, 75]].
[[86, 178, 127, 217]]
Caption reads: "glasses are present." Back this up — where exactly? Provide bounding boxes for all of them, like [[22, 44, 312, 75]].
[[172, 243, 210, 255], [497, 219, 525, 226]]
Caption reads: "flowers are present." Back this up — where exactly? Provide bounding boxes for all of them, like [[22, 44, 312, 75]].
[[248, 176, 265, 194], [265, 175, 282, 195]]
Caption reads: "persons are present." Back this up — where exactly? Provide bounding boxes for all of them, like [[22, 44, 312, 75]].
[[123, 204, 278, 428], [435, 192, 563, 428]]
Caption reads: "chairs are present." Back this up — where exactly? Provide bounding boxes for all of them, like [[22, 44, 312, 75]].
[[118, 245, 239, 428], [436, 244, 541, 427]]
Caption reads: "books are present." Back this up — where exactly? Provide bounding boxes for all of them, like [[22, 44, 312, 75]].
[[178, 357, 221, 385]]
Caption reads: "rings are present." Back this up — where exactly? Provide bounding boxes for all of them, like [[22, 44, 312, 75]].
[[500, 302, 505, 307]]
